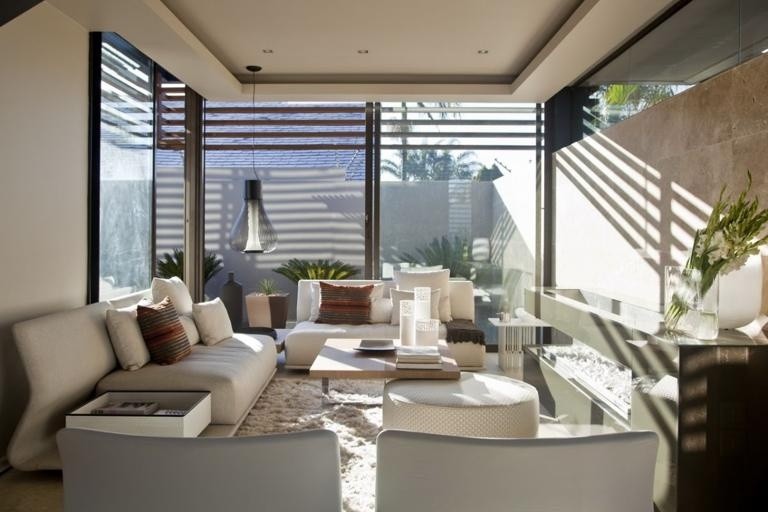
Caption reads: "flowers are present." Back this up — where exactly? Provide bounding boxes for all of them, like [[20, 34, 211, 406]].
[[664, 167, 768, 332]]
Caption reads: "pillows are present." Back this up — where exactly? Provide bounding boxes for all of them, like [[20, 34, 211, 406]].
[[395, 269, 454, 323], [309, 281, 386, 327], [315, 281, 376, 324], [177, 314, 202, 347], [135, 296, 193, 365], [370, 295, 450, 325], [103, 296, 155, 371], [150, 275, 194, 313], [389, 285, 442, 326], [193, 297, 234, 346]]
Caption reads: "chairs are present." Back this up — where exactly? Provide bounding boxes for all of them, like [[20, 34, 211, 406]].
[[55, 426, 344, 511], [374, 430, 660, 511]]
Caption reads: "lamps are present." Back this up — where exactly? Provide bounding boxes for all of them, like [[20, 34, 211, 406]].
[[227, 64, 279, 255]]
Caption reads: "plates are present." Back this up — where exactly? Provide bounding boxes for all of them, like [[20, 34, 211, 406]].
[[352, 338, 396, 351]]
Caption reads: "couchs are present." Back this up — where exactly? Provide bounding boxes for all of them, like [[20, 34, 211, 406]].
[[4, 275, 279, 474], [280, 278, 487, 372], [382, 371, 542, 440]]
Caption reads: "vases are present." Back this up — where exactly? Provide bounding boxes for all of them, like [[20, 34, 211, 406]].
[[664, 266, 720, 339], [221, 271, 245, 327]]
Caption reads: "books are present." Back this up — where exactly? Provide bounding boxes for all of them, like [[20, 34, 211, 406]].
[[90, 401, 160, 415], [396, 346, 443, 370]]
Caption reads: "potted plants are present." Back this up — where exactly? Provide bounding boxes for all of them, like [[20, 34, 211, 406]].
[[245, 277, 289, 331]]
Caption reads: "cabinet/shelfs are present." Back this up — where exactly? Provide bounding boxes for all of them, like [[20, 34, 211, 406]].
[[538, 287, 767, 511]]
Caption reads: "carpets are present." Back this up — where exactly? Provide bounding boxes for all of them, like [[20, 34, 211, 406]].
[[231, 374, 397, 511]]
